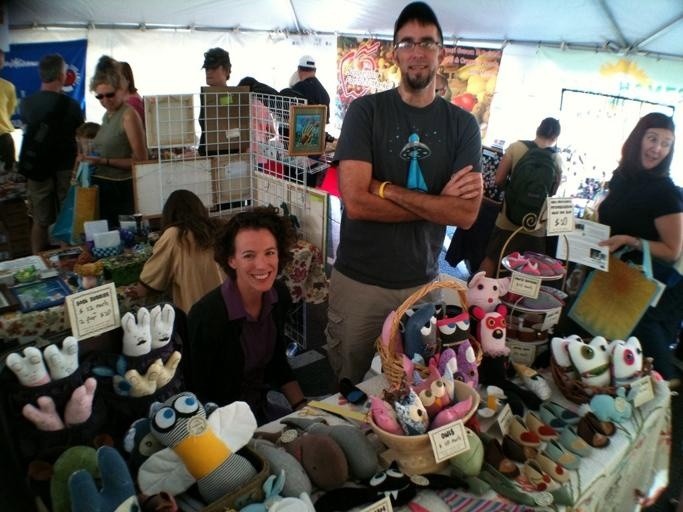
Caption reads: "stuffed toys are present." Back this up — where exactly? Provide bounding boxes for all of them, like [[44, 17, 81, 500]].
[[0, 273, 657, 512], [51, 392, 428, 512], [552, 335, 643, 422], [372, 271, 508, 436], [6, 336, 97, 429], [93, 304, 182, 397]]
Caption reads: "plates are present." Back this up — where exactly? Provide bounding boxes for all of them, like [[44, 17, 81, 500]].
[[501, 255, 564, 281], [501, 286, 568, 314]]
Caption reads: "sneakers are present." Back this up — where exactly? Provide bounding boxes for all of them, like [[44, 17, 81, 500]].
[[483, 402, 617, 508]]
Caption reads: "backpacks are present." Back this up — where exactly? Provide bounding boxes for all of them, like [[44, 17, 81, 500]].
[[507, 141, 560, 229]]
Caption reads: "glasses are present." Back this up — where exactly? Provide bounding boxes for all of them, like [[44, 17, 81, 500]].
[[391, 40, 443, 51], [96, 88, 117, 100]]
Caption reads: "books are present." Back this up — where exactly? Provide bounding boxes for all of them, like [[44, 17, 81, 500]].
[[556, 217, 614, 274]]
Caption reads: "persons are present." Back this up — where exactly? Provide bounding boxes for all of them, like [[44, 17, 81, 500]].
[[323, 0, 487, 389], [196, 45, 245, 211], [0, 45, 20, 172], [475, 114, 564, 280], [591, 109, 683, 385], [277, 54, 333, 188], [71, 119, 101, 185], [177, 207, 312, 424], [0, 131, 28, 265], [137, 187, 229, 319], [118, 60, 145, 125], [17, 50, 85, 254], [75, 53, 150, 230]]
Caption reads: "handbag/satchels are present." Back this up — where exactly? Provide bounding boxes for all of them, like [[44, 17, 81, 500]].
[[16, 96, 72, 181], [568, 236, 659, 345]]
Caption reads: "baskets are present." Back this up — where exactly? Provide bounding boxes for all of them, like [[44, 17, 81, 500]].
[[550, 338, 652, 405], [374, 280, 482, 390], [494, 212, 569, 358]]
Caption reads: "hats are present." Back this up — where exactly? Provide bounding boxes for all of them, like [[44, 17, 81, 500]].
[[202, 48, 229, 69], [299, 57, 317, 68], [393, 1, 444, 42]]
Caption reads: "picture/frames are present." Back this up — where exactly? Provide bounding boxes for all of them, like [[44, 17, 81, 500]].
[[200, 85, 248, 148], [131, 155, 217, 217], [10, 277, 73, 314], [0, 283, 20, 315], [290, 104, 327, 156], [252, 171, 328, 264], [142, 93, 195, 149]]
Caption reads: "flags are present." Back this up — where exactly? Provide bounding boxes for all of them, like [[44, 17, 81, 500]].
[[4, 40, 92, 134], [334, 34, 504, 144]]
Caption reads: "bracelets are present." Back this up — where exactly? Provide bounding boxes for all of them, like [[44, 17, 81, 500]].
[[377, 179, 392, 199], [631, 238, 641, 247], [291, 397, 309, 410], [106, 158, 110, 167]]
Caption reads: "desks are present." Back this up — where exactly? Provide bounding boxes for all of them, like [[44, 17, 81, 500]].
[[0, 209, 318, 407], [254, 370, 673, 512]]
